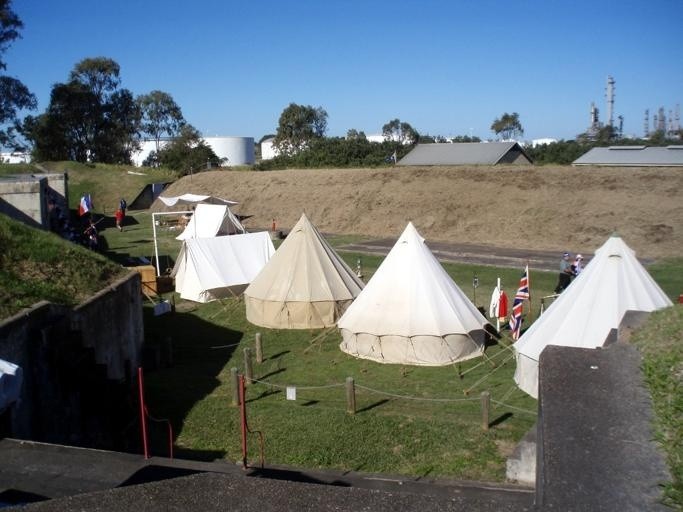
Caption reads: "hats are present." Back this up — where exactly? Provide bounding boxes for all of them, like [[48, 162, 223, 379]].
[[562, 252, 572, 257], [574, 254, 584, 261]]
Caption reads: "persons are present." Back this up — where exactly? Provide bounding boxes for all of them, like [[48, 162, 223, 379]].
[[114, 208, 123, 232], [119, 198, 126, 216], [48, 198, 96, 251], [553, 251, 571, 294], [572, 254, 584, 278]]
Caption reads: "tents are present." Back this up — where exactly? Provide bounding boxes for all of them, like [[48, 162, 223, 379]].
[[174, 203, 249, 241], [511, 228, 675, 402], [244, 213, 364, 330], [338, 220, 490, 367], [169, 227, 273, 303]]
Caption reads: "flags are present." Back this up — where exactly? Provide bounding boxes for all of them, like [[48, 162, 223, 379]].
[[78, 195, 89, 216], [489, 286, 498, 318], [508, 265, 528, 340], [498, 290, 507, 317]]
